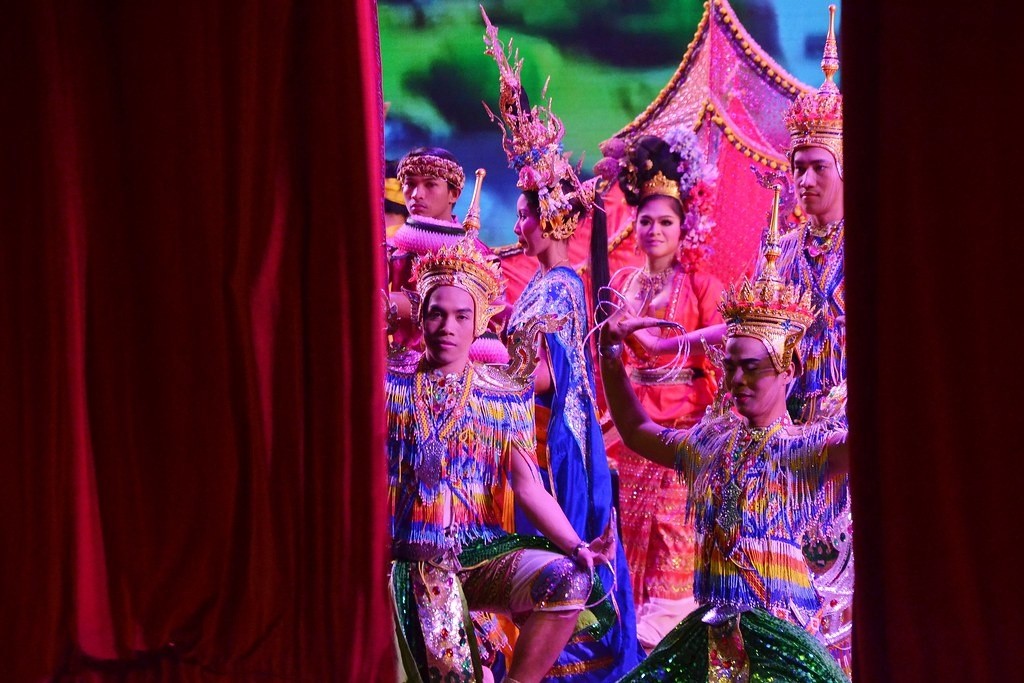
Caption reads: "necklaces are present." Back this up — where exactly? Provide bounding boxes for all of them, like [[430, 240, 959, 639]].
[[544, 258, 570, 278], [634, 265, 675, 300]]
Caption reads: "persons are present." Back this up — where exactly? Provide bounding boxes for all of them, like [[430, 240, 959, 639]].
[[386, 6, 852, 683]]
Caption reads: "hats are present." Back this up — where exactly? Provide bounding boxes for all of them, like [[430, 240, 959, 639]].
[[781, 4, 844, 182], [399, 168, 508, 341], [719, 183, 822, 375]]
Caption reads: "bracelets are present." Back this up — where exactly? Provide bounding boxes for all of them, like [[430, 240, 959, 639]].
[[571, 541, 587, 561], [598, 343, 622, 358]]
